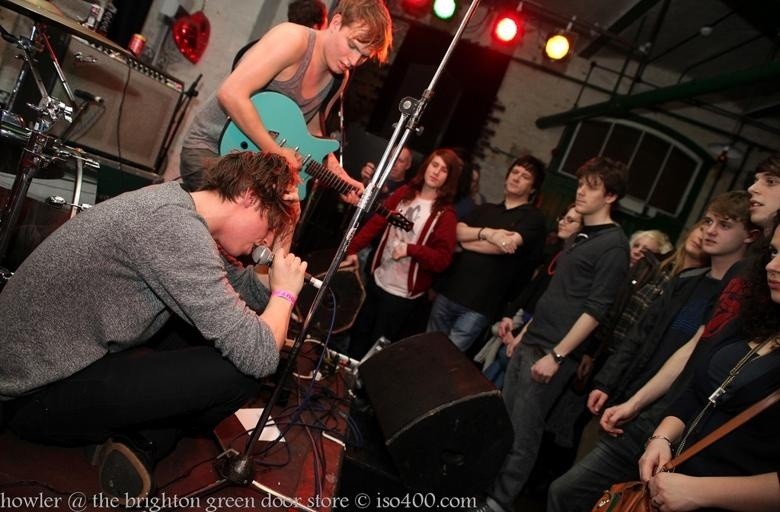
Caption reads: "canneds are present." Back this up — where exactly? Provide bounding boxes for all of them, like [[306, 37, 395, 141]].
[[126, 33, 146, 58], [80, 3, 104, 32]]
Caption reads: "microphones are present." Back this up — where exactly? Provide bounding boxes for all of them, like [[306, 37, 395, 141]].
[[73, 86, 102, 105], [254, 245, 321, 288]]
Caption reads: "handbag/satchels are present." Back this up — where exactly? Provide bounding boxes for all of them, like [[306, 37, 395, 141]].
[[590, 477, 659, 512]]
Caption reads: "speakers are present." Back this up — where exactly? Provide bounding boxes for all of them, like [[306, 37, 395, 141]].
[[1, 4, 32, 113], [35, 34, 180, 173], [358, 331, 514, 483]]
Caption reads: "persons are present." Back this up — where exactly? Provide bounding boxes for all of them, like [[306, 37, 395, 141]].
[[0, 1, 779, 510]]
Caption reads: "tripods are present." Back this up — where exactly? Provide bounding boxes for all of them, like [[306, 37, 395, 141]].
[[158, 1, 483, 511]]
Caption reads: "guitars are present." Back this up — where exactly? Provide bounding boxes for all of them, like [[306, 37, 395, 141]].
[[218, 89, 414, 232]]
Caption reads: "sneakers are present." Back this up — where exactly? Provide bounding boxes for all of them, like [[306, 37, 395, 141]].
[[82, 427, 159, 508], [467, 502, 501, 512]]
